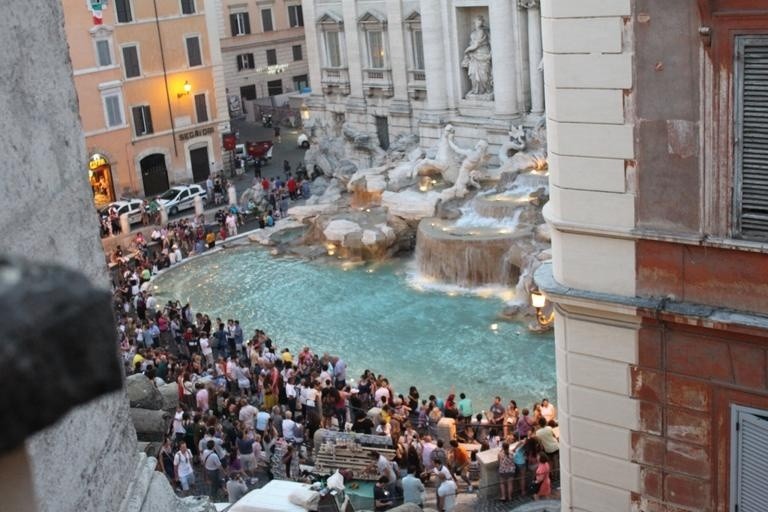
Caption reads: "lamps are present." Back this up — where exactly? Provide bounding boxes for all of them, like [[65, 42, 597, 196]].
[[528, 286, 555, 327]]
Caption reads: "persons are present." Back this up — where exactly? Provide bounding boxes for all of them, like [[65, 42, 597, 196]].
[[108, 280, 560, 512], [463, 16, 493, 95], [99, 156, 323, 287], [447, 134, 489, 191], [273, 123, 281, 143]]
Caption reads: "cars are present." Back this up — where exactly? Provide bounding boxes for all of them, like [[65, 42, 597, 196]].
[[296, 132, 310, 149], [145, 182, 208, 217], [99, 196, 146, 229]]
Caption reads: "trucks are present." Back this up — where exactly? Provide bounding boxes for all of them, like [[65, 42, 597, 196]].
[[235, 141, 274, 166]]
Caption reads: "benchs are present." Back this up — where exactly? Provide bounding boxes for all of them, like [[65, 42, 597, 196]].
[[311, 440, 398, 482]]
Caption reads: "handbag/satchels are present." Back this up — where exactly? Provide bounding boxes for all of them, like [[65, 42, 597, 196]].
[[529, 479, 543, 495]]
